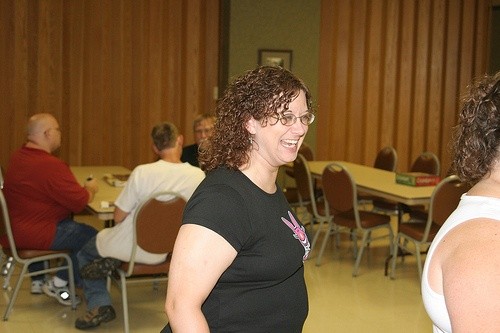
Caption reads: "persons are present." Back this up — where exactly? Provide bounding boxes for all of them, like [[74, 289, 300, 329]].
[[421, 72, 500, 333], [160, 65, 315, 333], [75, 122, 206, 330], [179, 114, 220, 169], [0, 113, 100, 306]]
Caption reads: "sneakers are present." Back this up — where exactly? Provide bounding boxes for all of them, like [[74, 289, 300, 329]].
[[82, 258, 115, 279], [41, 277, 83, 305], [75, 305, 116, 330], [30, 279, 44, 293]]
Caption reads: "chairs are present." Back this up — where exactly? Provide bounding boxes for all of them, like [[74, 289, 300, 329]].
[[349, 148, 398, 246], [390, 175, 473, 280], [373, 152, 440, 214], [106, 191, 187, 333], [315, 163, 393, 279], [0, 169, 77, 321], [292, 154, 357, 261]]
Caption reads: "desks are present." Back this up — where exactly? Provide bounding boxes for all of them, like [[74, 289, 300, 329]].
[[69, 165, 133, 228], [283, 158, 436, 277]]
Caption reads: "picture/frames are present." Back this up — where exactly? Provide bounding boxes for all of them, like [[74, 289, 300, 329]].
[[258, 48, 293, 73]]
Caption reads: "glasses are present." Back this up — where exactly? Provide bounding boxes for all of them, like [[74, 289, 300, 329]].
[[266, 112, 316, 126]]
